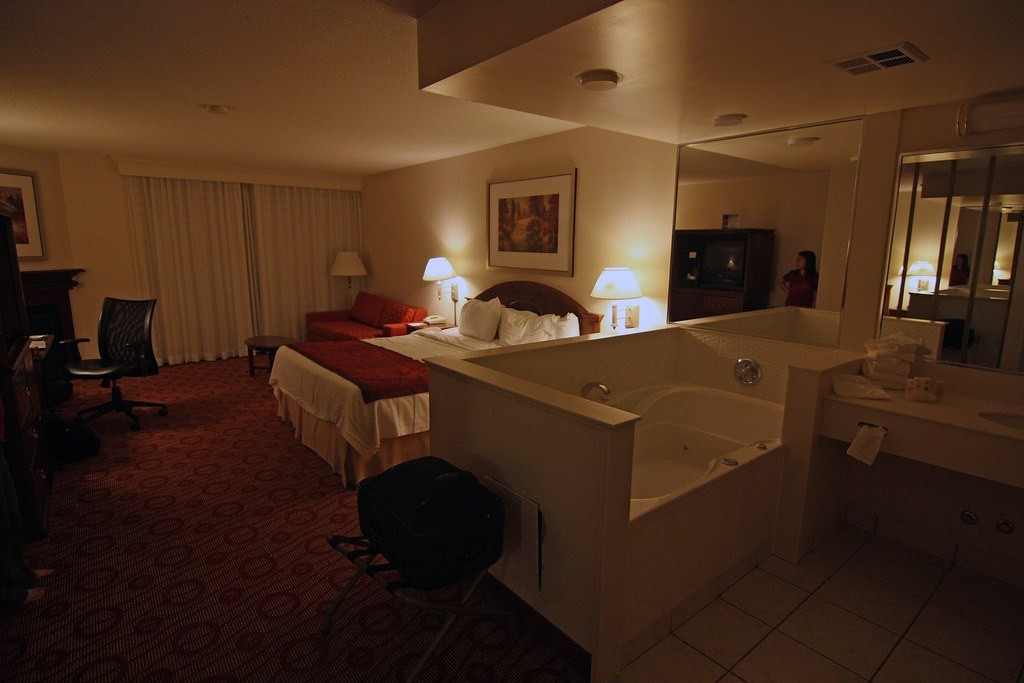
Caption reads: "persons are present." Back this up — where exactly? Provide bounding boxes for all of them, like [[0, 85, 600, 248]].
[[948, 254, 971, 287], [777, 249, 818, 309]]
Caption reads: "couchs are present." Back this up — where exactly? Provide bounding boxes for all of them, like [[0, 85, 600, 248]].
[[306, 291, 427, 341]]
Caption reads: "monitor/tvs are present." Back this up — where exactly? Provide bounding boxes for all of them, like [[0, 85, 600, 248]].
[[699, 241, 745, 290], [0, 213, 31, 378]]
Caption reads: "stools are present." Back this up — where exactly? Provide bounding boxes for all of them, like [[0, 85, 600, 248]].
[[323, 535, 530, 683], [245, 335, 291, 377]]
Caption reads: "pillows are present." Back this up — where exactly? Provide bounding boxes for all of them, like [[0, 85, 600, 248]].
[[459, 296, 579, 347]]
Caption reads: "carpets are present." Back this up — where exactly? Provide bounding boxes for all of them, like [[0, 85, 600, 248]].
[[0, 355, 591, 683]]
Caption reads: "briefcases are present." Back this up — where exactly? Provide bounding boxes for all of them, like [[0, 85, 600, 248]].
[[356, 457, 505, 590]]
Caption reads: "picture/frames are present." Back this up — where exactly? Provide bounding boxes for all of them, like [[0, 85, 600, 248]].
[[486, 168, 576, 276], [0, 167, 48, 261]]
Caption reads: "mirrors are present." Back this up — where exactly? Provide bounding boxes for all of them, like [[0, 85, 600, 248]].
[[875, 141, 1024, 376]]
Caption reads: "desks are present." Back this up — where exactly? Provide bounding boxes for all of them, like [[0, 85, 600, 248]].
[[20, 269, 85, 366], [29, 335, 55, 360]]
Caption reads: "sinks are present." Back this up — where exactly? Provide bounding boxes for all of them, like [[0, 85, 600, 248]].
[[978, 412, 1024, 430]]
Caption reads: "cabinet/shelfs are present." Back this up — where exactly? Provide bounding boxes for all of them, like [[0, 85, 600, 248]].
[[671, 228, 774, 322], [0, 201, 52, 540]]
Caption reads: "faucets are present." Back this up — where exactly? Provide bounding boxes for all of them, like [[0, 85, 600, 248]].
[[576, 381, 611, 398]]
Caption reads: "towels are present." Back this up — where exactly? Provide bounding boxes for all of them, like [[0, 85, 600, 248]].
[[832, 332, 924, 400], [845, 423, 889, 467]]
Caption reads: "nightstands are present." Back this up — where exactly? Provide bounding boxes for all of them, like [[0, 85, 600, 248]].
[[405, 322, 458, 335]]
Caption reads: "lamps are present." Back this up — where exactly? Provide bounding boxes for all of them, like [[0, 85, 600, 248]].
[[330, 252, 368, 295], [422, 257, 458, 302], [590, 268, 642, 330]]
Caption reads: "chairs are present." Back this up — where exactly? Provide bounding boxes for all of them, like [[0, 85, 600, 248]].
[[58, 297, 169, 435]]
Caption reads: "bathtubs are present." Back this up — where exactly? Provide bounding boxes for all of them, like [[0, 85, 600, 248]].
[[560, 379, 786, 646]]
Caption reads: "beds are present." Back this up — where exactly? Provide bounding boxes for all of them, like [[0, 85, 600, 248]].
[[269, 281, 604, 491]]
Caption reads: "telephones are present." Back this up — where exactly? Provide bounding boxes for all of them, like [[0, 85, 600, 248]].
[[424, 315, 446, 324]]
